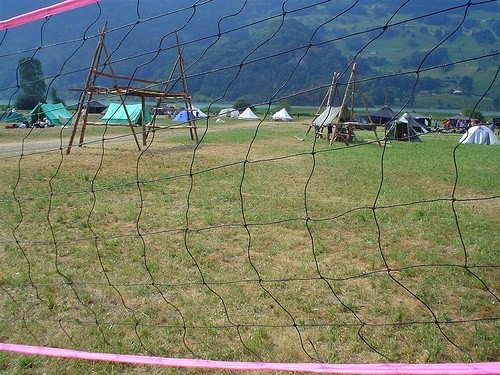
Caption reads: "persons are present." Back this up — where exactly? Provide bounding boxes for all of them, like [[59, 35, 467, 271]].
[[81, 106, 87, 121], [43, 118, 50, 127]]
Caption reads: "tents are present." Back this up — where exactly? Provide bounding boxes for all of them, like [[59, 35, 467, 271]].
[[102, 102, 152, 125], [313, 104, 356, 127], [172, 111, 195, 123], [272, 108, 293, 121], [27, 101, 72, 126], [459, 126, 500, 145], [396, 112, 427, 134], [238, 107, 258, 119], [383, 118, 423, 143]]
